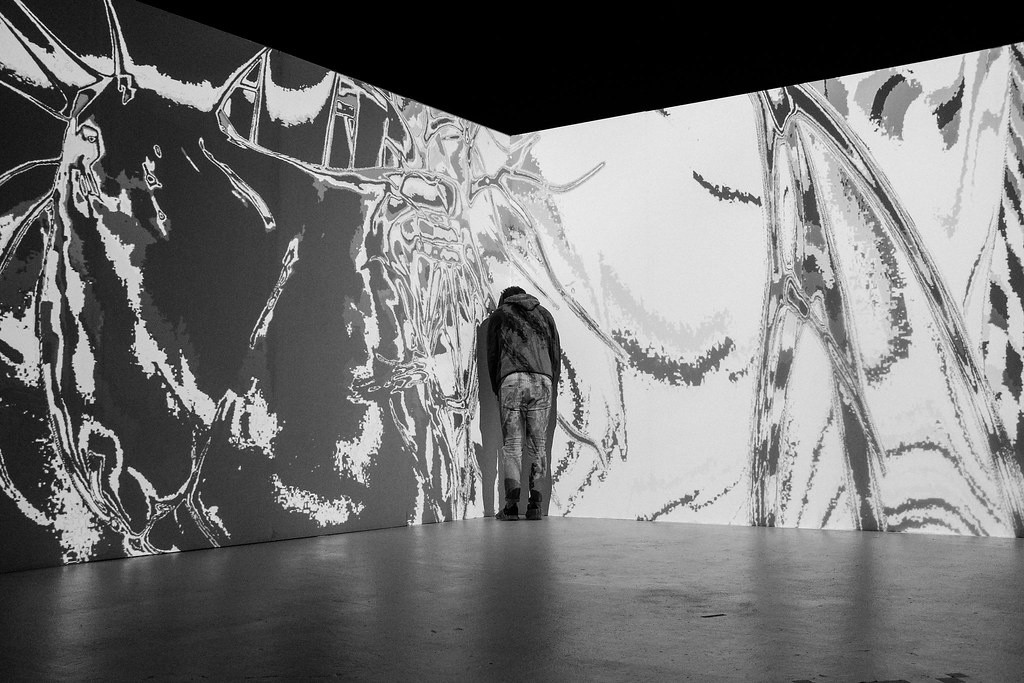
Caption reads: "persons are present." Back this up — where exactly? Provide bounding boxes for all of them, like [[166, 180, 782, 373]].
[[487, 286, 560, 520]]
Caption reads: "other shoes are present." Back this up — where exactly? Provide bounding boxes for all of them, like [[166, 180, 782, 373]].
[[496, 502, 543, 520]]
